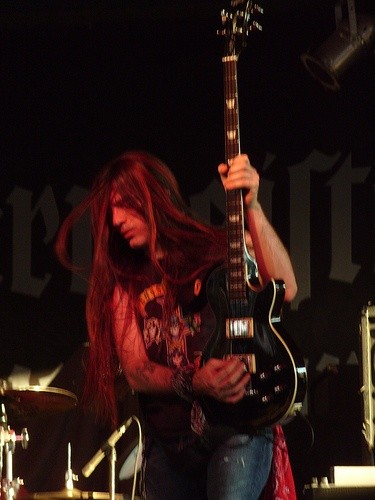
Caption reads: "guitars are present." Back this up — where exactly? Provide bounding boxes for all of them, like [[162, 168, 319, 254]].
[[191, 0, 311, 437]]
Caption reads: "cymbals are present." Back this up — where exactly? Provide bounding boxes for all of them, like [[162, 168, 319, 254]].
[[0, 384, 80, 416]]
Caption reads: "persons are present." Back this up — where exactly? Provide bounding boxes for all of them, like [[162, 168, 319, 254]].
[[86, 152, 299, 499]]
